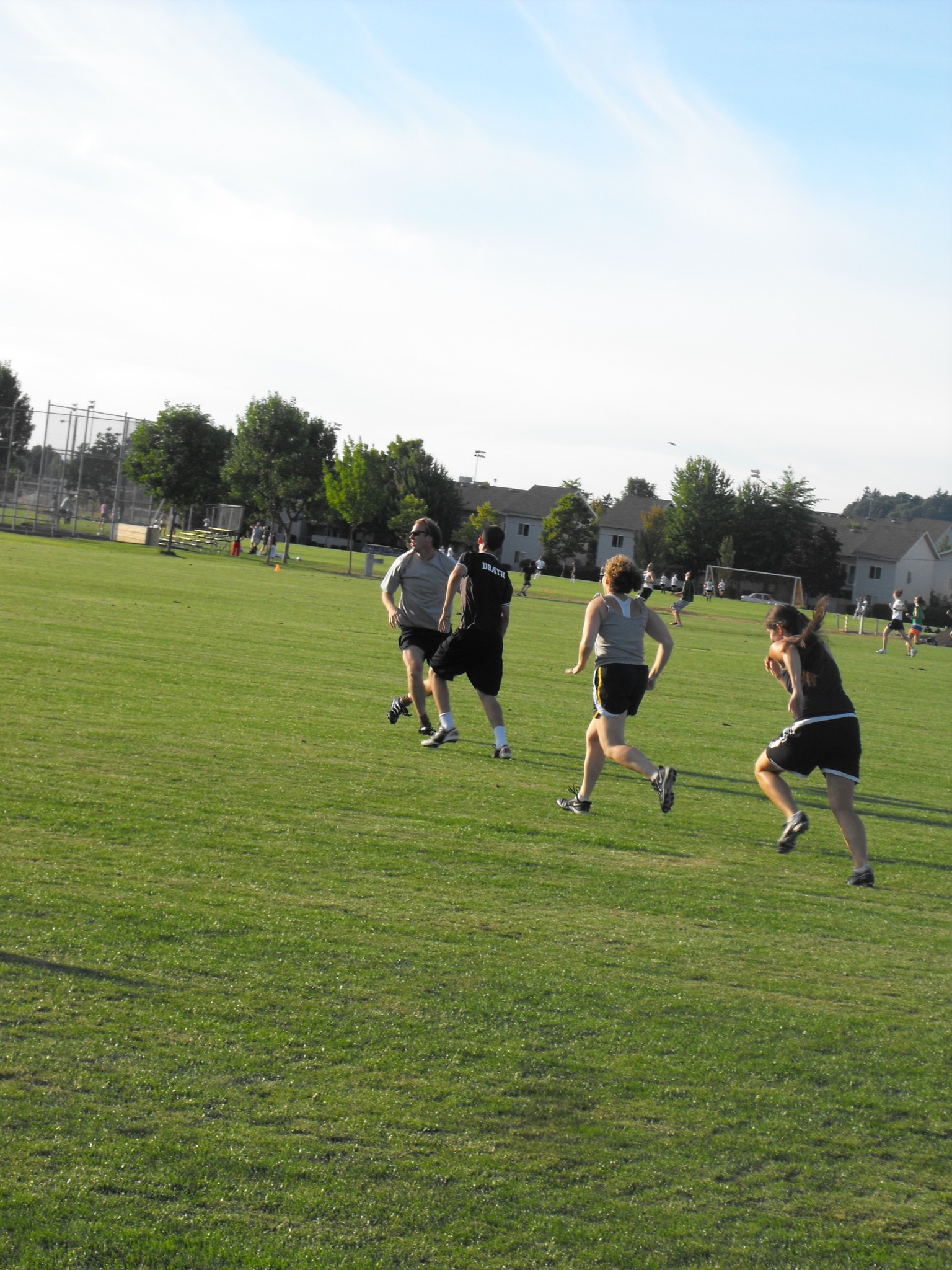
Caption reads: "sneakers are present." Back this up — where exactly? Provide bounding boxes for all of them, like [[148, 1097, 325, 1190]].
[[557, 784, 592, 815], [490, 744, 511, 760], [421, 726, 459, 746], [847, 865, 874, 888], [910, 648, 918, 658], [651, 765, 676, 813], [775, 810, 809, 855], [388, 697, 412, 724], [419, 725, 436, 737], [876, 649, 886, 654]]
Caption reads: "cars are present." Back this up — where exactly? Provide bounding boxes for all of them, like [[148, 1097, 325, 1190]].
[[740, 592, 784, 606]]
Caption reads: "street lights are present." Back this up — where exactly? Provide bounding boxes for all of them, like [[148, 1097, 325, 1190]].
[[89, 400, 96, 449], [60, 418, 78, 461], [71, 405, 96, 535], [473, 450, 487, 482]]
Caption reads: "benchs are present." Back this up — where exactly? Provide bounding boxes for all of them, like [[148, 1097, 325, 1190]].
[[159, 527, 237, 556]]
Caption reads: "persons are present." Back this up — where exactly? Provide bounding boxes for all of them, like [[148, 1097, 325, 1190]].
[[598, 564, 605, 583], [50, 493, 78, 524], [635, 563, 655, 603], [97, 499, 124, 529], [446, 544, 454, 558], [534, 556, 545, 579], [156, 509, 180, 536], [570, 563, 576, 584], [852, 597, 868, 621], [438, 545, 445, 554], [248, 521, 277, 563], [231, 530, 244, 556], [670, 571, 694, 627], [660, 573, 667, 594], [421, 525, 513, 760], [378, 519, 463, 736], [556, 553, 677, 814], [516, 560, 537, 597], [718, 578, 725, 599], [905, 596, 939, 657], [671, 573, 678, 595], [703, 578, 714, 602], [755, 596, 876, 885], [202, 516, 210, 529], [875, 589, 917, 658], [945, 610, 952, 638]]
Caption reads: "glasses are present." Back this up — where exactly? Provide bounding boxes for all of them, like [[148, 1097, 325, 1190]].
[[410, 530, 428, 536]]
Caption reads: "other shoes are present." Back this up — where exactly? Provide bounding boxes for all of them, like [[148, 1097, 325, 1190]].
[[517, 593, 521, 596], [675, 624, 682, 627], [522, 591, 526, 596], [927, 638, 938, 647], [669, 620, 677, 625]]
